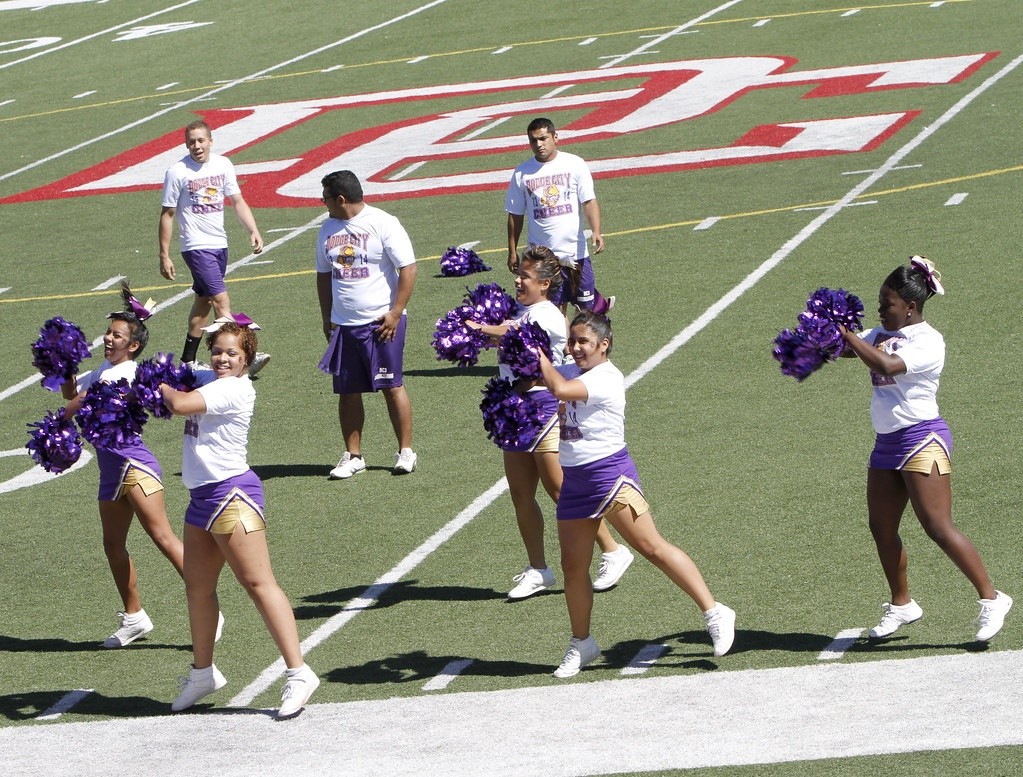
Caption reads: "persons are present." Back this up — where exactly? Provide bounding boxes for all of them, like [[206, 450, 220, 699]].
[[58, 311, 225, 646], [159, 122, 270, 377], [465, 244, 635, 598], [316, 170, 417, 479], [839, 256, 1014, 642], [159, 320, 322, 716], [505, 118, 604, 364], [537, 309, 735, 678]]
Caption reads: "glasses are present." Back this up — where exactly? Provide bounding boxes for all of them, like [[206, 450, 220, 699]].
[[320, 194, 342, 204]]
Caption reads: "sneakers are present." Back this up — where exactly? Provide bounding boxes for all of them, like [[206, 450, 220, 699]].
[[248, 351, 270, 376], [394, 447, 418, 474], [704, 601, 736, 657], [179, 358, 212, 371], [330, 451, 367, 479], [507, 564, 556, 600], [868, 598, 923, 638], [215, 610, 224, 642], [592, 543, 634, 590], [278, 662, 320, 716], [976, 591, 1013, 640], [171, 663, 227, 711], [553, 633, 600, 678], [103, 609, 153, 647]]
